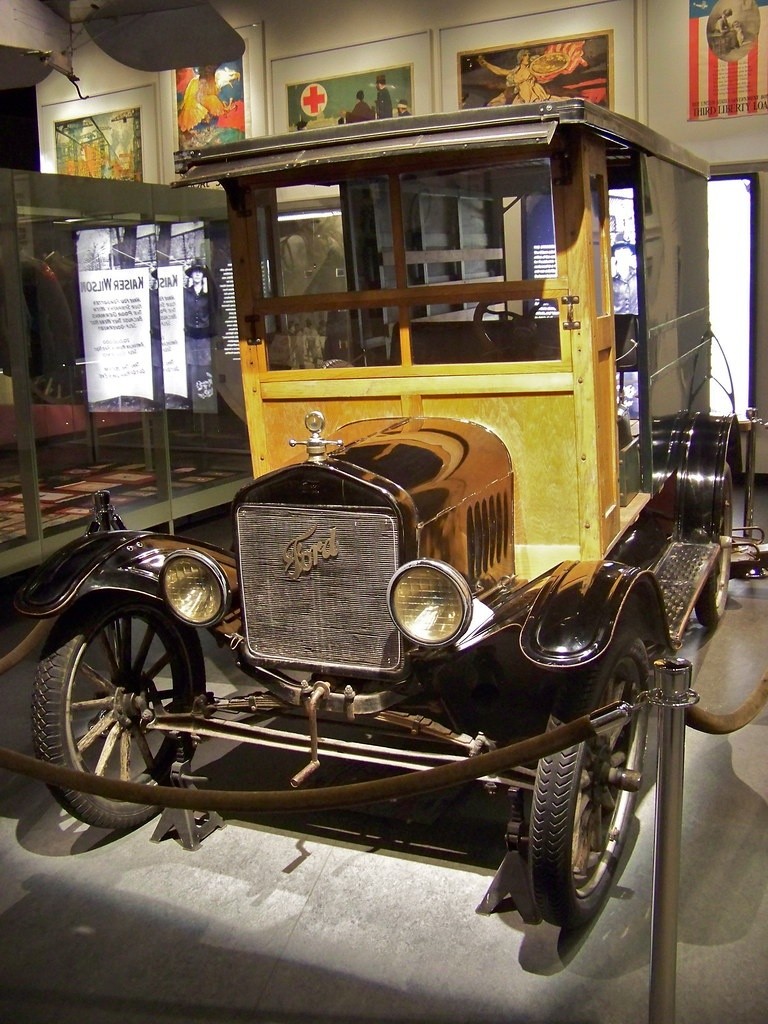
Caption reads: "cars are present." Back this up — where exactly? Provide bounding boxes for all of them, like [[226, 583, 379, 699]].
[[10, 97, 737, 934]]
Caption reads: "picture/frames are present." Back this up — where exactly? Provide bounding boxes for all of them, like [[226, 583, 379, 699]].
[[268, 28, 434, 133], [40, 82, 161, 184], [438, 0, 639, 123], [168, 21, 267, 188]]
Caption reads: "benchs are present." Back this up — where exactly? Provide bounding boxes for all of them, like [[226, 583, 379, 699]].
[[390, 314, 639, 508]]
